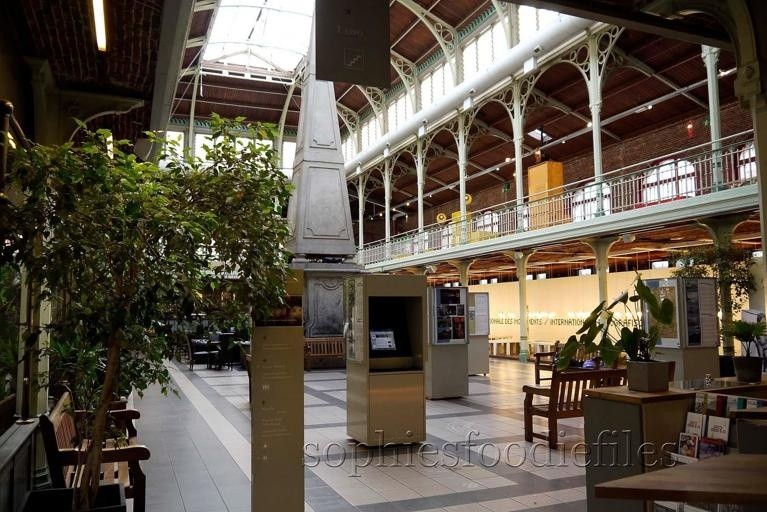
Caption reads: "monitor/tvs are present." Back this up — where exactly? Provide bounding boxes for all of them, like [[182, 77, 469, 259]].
[[369, 325, 397, 352]]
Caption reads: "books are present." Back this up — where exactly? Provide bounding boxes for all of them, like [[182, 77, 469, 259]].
[[676, 389, 767, 461]]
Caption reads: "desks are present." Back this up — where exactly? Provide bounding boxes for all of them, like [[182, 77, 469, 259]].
[[593, 454, 767, 512], [581, 372, 767, 512]]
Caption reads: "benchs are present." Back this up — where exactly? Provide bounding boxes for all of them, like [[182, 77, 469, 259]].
[[522, 341, 677, 449]]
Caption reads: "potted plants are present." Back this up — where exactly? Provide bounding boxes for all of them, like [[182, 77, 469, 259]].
[[721, 320, 767, 383], [0, 112, 299, 512], [554, 271, 676, 394]]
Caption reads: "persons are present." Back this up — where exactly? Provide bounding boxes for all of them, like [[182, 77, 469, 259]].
[[752, 312, 767, 372]]
